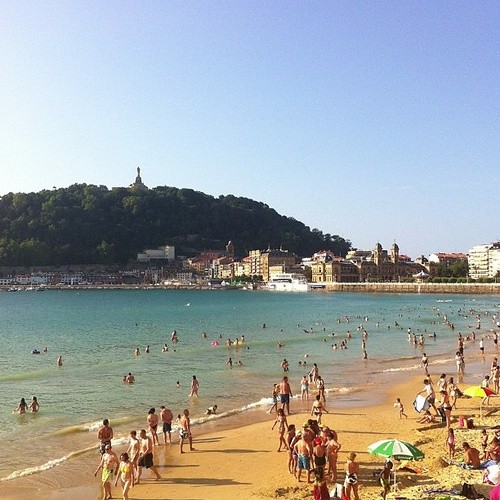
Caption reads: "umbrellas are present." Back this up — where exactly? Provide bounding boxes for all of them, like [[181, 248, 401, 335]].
[[462, 385, 497, 408], [480, 386, 497, 397], [368, 438, 426, 493]]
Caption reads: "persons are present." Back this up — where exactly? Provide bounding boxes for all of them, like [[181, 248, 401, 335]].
[[57, 356, 62, 368], [131, 429, 161, 484], [176, 380, 180, 385], [114, 452, 134, 500], [175, 409, 196, 454], [134, 322, 250, 370], [159, 406, 174, 444], [13, 396, 40, 414], [276, 377, 293, 416], [93, 444, 120, 500], [123, 372, 136, 383], [294, 431, 314, 483], [261, 298, 500, 500], [271, 409, 289, 453], [203, 405, 218, 415], [189, 374, 200, 398], [125, 430, 140, 480], [97, 418, 113, 470], [343, 453, 361, 500], [32, 347, 48, 353], [147, 408, 160, 447], [437, 390, 451, 432]]
